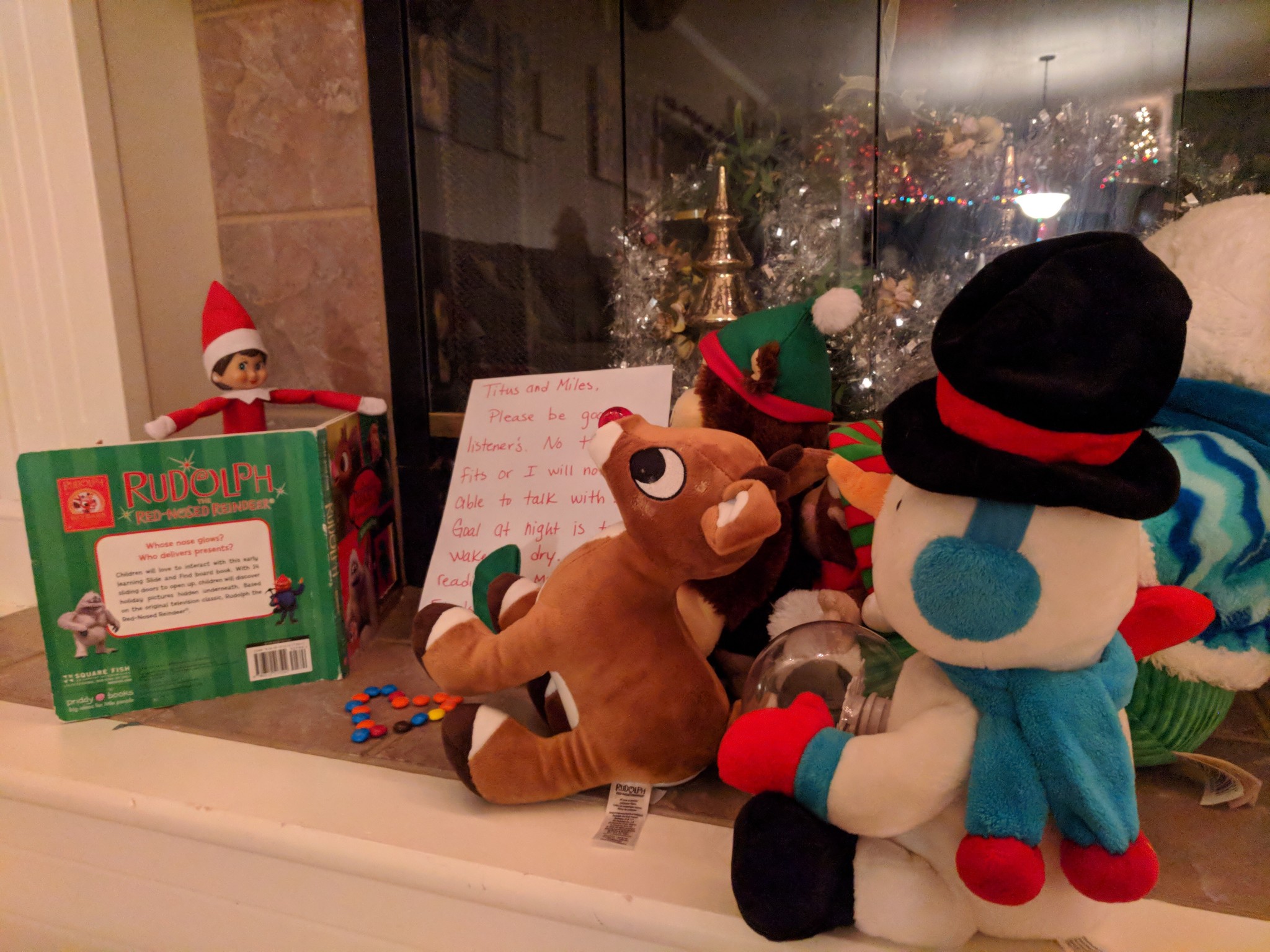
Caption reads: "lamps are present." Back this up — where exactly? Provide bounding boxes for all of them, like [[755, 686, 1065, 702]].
[[1014, 55, 1071, 219]]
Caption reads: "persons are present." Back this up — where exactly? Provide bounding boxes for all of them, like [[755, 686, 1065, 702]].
[[144, 279, 388, 441]]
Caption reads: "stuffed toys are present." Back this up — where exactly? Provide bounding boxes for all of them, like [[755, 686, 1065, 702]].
[[667, 193, 1270, 769], [720, 230, 1213, 942], [408, 404, 838, 806]]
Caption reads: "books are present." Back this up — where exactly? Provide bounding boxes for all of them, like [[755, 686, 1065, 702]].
[[16, 410, 403, 724]]
